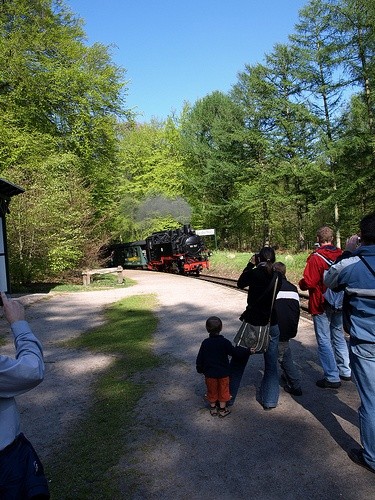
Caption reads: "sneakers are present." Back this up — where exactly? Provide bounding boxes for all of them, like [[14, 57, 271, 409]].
[[219, 408, 231, 418], [210, 407, 218, 417]]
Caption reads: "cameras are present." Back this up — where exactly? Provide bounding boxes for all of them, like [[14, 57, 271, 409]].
[[315, 243, 320, 248], [358, 236, 361, 243], [0, 297, 3, 306], [255, 254, 260, 263]]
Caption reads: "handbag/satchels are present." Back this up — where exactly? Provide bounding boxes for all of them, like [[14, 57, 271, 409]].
[[234, 320, 270, 354]]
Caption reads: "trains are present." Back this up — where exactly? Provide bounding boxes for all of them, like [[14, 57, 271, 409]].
[[98, 224, 212, 277]]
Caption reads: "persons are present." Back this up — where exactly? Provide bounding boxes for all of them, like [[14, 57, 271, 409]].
[[196, 316, 256, 418], [215, 247, 283, 410], [299, 227, 352, 389], [271, 262, 304, 397], [0, 292, 50, 500], [323, 211, 375, 474]]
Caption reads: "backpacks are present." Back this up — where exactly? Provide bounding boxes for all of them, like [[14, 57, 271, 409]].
[[314, 252, 344, 311]]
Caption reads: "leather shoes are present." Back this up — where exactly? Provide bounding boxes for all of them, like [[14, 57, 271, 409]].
[[283, 385, 303, 396], [350, 448, 375, 474], [339, 375, 351, 381], [316, 378, 342, 388]]
